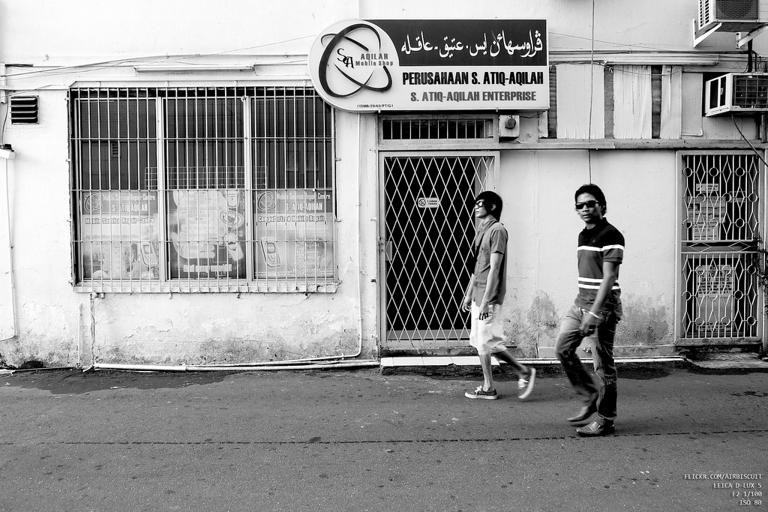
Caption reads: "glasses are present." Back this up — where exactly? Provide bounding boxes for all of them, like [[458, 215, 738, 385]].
[[576, 200, 599, 210], [474, 201, 484, 208]]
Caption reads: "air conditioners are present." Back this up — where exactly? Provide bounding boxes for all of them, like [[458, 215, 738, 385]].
[[698, 1, 768, 33]]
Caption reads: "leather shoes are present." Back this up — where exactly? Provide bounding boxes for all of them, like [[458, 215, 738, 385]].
[[567, 397, 598, 422], [576, 416, 615, 436]]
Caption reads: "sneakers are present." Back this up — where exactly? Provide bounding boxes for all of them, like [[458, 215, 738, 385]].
[[464, 387, 498, 401], [518, 365, 537, 400]]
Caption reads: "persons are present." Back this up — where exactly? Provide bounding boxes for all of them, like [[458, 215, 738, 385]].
[[554, 183, 626, 438], [460, 191, 536, 401]]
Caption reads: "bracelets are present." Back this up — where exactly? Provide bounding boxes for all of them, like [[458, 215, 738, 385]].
[[588, 310, 602, 320]]
